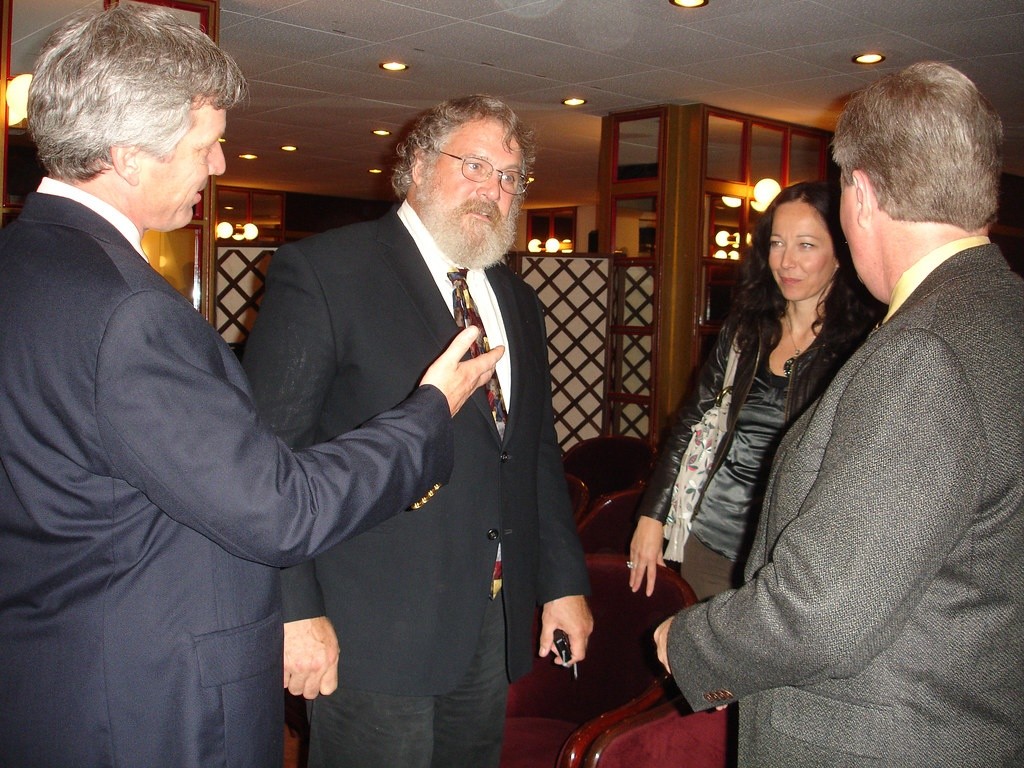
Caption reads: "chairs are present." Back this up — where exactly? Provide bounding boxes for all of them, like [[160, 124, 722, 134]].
[[495, 435, 737, 767]]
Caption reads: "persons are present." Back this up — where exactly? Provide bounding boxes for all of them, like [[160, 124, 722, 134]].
[[242, 93, 594, 768], [629, 182, 888, 603], [1, 0, 506, 768], [656, 59, 1024, 768]]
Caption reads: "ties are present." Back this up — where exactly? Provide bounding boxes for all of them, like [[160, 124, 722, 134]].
[[447, 267, 508, 601]]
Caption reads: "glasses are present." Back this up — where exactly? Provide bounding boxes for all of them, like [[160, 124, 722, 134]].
[[439, 150, 530, 195]]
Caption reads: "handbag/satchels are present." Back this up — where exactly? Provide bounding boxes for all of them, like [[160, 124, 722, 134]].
[[661, 324, 750, 563]]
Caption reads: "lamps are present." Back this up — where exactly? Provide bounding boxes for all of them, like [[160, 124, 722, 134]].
[[752, 177, 782, 203], [4, 72, 34, 110], [218, 222, 258, 240], [526, 238, 563, 253], [715, 231, 740, 247]]
